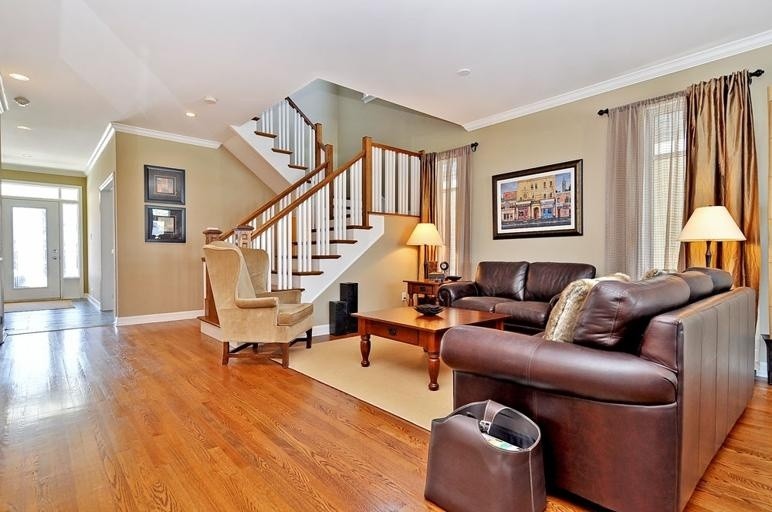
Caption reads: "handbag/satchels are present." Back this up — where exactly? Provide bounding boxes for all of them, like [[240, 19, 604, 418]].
[[424, 400, 546, 512]]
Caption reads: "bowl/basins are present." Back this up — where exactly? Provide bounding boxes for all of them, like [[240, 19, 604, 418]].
[[447, 276, 462, 281]]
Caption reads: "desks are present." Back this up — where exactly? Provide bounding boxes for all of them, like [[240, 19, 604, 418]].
[[403, 280, 453, 306]]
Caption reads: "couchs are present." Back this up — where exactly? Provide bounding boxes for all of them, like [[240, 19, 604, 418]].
[[438, 261, 596, 332], [440, 287, 756, 512]]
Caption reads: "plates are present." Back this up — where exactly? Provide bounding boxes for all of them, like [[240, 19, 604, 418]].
[[414, 303, 445, 316]]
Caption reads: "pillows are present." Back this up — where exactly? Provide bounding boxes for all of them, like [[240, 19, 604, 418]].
[[476, 259, 734, 343]]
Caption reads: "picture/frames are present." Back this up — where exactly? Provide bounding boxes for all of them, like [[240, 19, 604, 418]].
[[492, 159, 583, 240], [144, 205, 186, 243], [144, 164, 185, 205]]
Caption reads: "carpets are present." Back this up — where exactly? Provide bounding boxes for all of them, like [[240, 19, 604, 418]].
[[4, 300, 75, 314], [270, 334, 453, 432]]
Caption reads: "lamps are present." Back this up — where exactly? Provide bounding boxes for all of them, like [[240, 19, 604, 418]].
[[676, 206, 747, 267], [406, 223, 443, 279]]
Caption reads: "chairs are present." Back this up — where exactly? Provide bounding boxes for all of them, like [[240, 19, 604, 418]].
[[202, 240, 315, 369]]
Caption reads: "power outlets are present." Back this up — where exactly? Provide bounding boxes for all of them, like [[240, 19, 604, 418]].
[[402, 292, 407, 301]]
